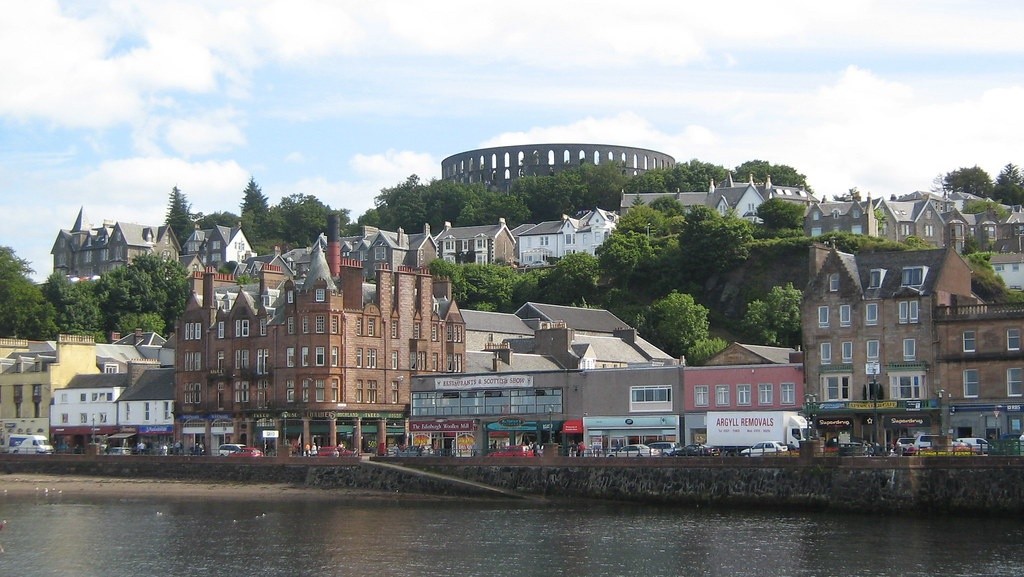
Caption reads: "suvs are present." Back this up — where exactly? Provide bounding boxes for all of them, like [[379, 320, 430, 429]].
[[218, 444, 246, 457]]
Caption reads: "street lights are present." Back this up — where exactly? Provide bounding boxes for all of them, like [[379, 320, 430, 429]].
[[548, 407, 553, 444], [283, 412, 289, 446], [92, 413, 96, 445], [993, 410, 1001, 439], [352, 424, 356, 445], [804, 393, 812, 442]]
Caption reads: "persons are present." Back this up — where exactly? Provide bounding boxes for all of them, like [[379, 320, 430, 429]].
[[579, 443, 585, 457], [137, 432, 1024, 460], [571, 440, 578, 458]]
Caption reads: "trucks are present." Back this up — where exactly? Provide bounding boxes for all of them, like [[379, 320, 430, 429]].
[[706, 411, 812, 457]]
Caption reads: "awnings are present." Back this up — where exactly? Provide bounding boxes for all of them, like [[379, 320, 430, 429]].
[[138, 431, 172, 435], [108, 433, 136, 439]]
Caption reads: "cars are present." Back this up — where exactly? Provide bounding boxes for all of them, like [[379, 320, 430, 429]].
[[739, 441, 788, 458], [670, 444, 720, 456], [227, 448, 263, 458], [486, 444, 534, 457], [952, 442, 977, 453], [606, 444, 650, 458], [317, 446, 353, 457], [894, 434, 940, 455]]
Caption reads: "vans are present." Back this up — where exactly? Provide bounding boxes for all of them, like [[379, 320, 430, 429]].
[[9, 435, 55, 454], [647, 441, 678, 456], [956, 437, 994, 454]]
